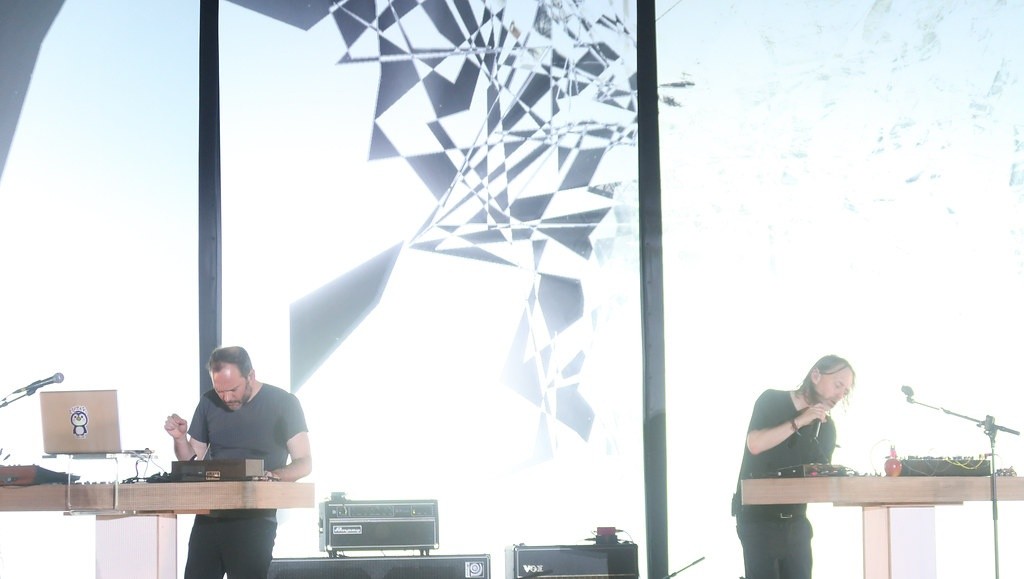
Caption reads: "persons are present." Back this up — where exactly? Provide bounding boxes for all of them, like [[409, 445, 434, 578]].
[[164, 346, 312, 579], [732, 355, 855, 579]]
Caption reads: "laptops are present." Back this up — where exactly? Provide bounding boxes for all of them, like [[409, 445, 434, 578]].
[[41, 389, 156, 455]]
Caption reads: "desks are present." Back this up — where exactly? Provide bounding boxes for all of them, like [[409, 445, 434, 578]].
[[0, 481, 315, 579], [739, 476, 1024, 579]]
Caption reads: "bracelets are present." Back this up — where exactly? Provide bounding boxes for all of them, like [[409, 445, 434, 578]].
[[790, 418, 799, 432]]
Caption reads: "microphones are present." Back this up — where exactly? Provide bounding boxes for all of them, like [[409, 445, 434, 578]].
[[13, 373, 65, 393], [810, 420, 822, 440]]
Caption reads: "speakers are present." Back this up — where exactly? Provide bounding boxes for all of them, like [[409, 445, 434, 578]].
[[266, 554, 491, 579], [505, 545, 639, 579]]
